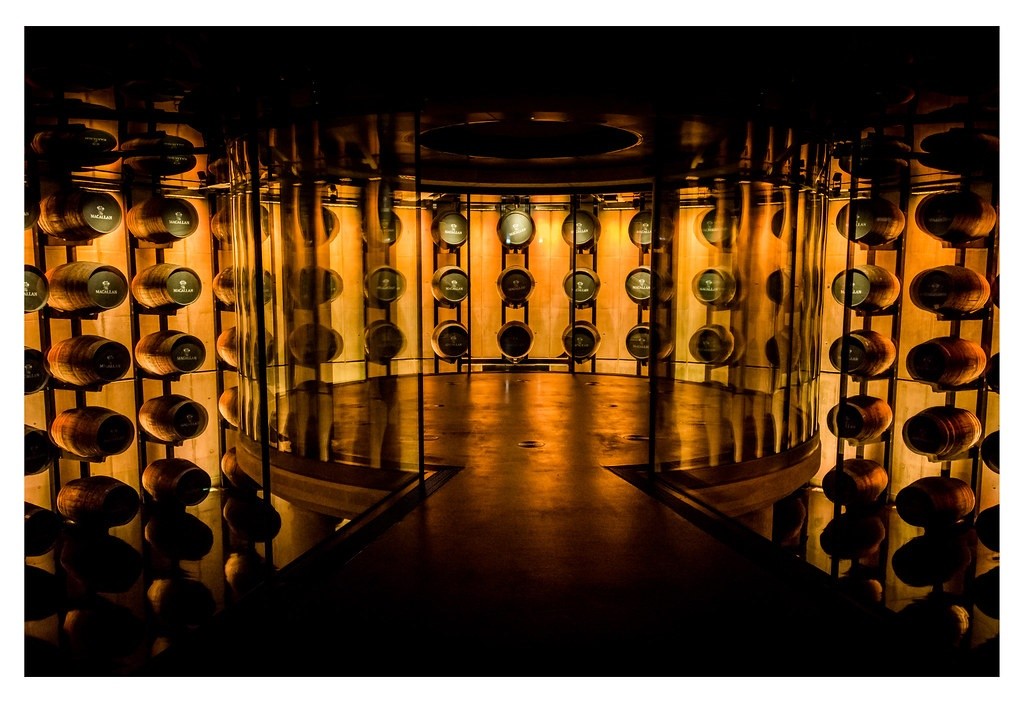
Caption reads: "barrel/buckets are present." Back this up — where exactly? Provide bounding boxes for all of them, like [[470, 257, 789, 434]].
[[25, 124, 998, 678], [25, 25, 999, 677]]
